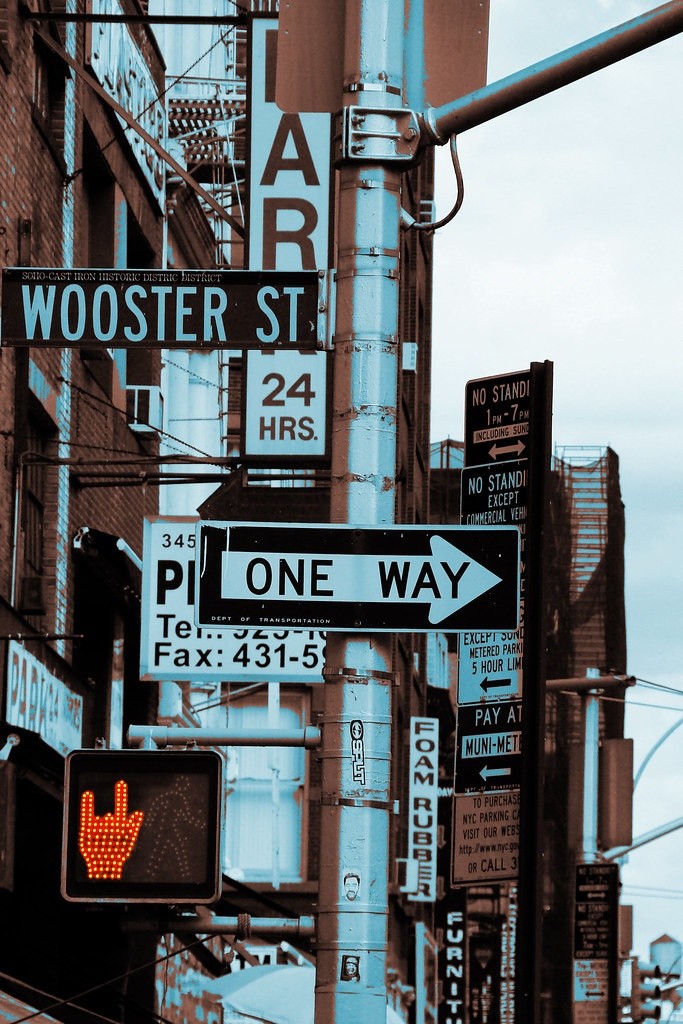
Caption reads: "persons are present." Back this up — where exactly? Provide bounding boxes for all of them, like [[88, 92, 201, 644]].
[[340, 958, 359, 982], [341, 873, 361, 901]]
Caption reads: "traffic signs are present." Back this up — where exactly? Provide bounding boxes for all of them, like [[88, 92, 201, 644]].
[[193, 524, 520, 632], [454, 706, 521, 798], [455, 632, 522, 705], [463, 368, 529, 468], [458, 471, 528, 600]]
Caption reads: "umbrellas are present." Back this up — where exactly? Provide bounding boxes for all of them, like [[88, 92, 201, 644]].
[[206, 965, 403, 1024]]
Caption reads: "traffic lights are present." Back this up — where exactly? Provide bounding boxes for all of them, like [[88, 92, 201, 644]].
[[61, 746, 223, 904], [630, 961, 663, 1022]]
